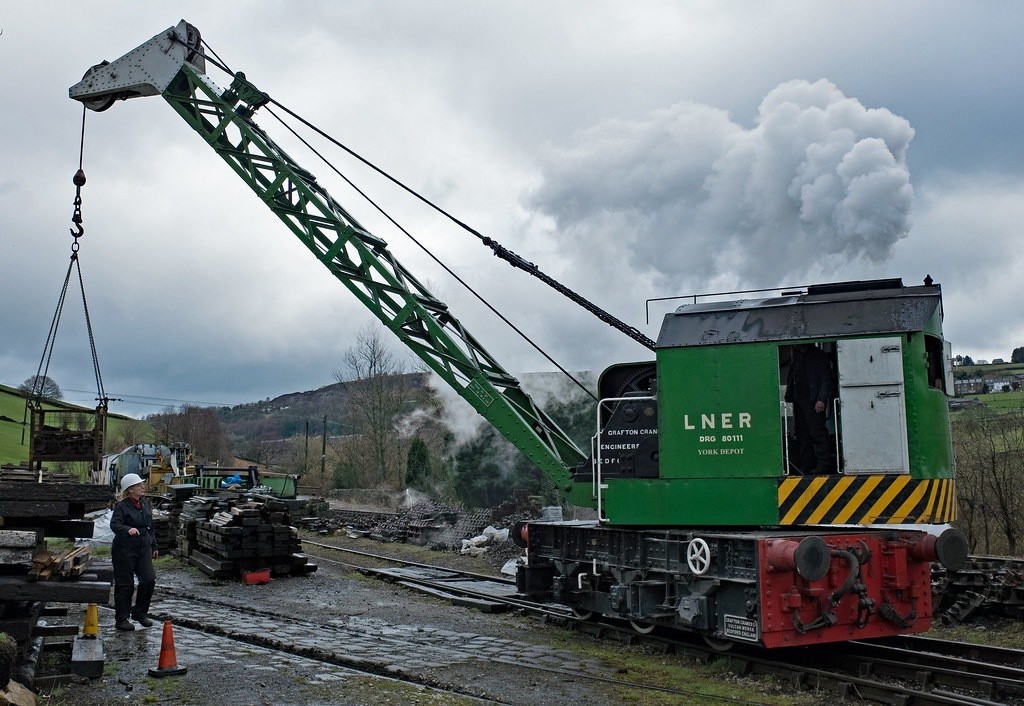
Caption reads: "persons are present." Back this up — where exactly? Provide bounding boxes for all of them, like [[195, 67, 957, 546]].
[[109, 473, 159, 631], [784, 343, 833, 475]]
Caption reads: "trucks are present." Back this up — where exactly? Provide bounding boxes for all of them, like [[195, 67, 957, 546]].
[[109, 442, 300, 502]]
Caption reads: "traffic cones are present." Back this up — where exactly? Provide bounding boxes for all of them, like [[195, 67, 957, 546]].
[[78, 603, 102, 641], [148, 620, 187, 678]]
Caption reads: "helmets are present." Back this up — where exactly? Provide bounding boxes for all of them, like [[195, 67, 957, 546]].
[[119, 472, 146, 492]]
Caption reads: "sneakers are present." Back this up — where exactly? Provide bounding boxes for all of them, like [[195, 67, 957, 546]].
[[132, 612, 154, 627], [115, 620, 134, 631]]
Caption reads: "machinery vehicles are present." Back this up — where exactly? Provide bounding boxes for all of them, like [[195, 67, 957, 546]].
[[68, 19, 969, 653]]
